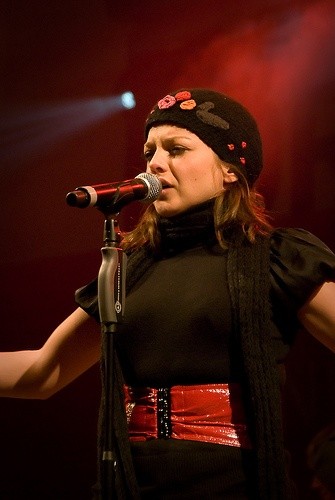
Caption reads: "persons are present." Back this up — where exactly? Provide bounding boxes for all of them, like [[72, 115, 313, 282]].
[[0, 88, 335, 500], [305, 429, 335, 500]]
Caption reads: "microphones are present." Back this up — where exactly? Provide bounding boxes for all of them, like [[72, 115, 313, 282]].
[[64, 173, 161, 209]]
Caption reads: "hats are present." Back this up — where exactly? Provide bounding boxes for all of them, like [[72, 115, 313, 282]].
[[145, 89, 263, 190]]
